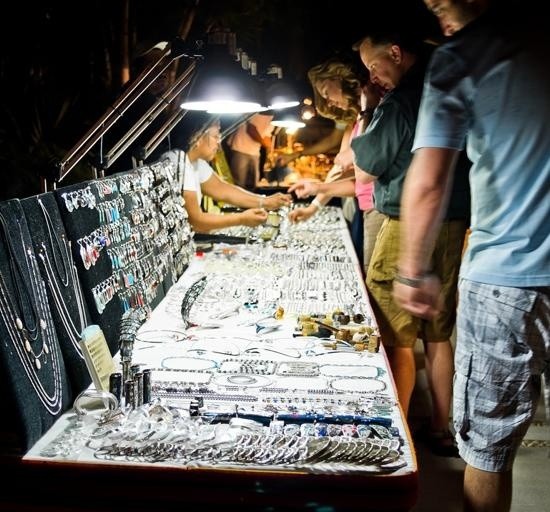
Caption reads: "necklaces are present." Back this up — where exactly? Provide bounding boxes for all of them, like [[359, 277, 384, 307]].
[[0, 195, 89, 418]]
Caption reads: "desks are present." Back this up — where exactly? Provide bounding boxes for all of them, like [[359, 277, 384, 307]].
[[15, 194, 422, 512]]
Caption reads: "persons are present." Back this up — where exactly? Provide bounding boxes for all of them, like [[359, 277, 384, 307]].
[[156, 117, 292, 234], [350, 22, 460, 459], [225, 112, 274, 192], [273, 118, 348, 168], [285, 71, 389, 276], [392, 2, 549, 509], [288, 62, 364, 273]]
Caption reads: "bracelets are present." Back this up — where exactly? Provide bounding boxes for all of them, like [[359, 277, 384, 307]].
[[92, 202, 408, 472], [310, 198, 325, 212], [257, 194, 267, 209], [393, 273, 428, 289]]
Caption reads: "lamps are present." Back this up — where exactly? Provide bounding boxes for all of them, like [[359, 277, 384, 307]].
[[90, 45, 316, 178], [37, 22, 266, 193]]
